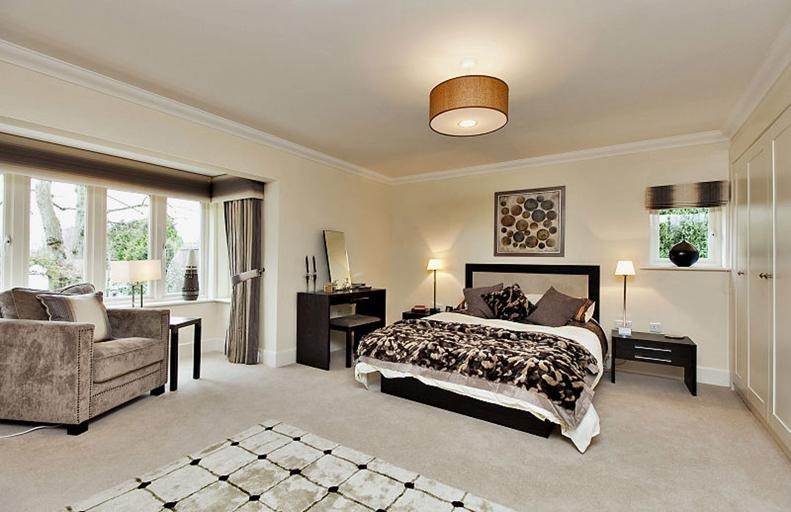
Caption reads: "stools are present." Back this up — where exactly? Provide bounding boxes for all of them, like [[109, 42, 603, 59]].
[[330, 314, 381, 368]]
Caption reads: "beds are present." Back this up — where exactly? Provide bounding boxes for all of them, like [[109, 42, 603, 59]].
[[357, 262, 609, 453]]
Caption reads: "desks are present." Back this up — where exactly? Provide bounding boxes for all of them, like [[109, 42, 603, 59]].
[[297, 288, 385, 371]]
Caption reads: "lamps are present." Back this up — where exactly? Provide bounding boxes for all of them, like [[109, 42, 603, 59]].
[[109, 261, 136, 308], [428, 76, 510, 137], [132, 259, 161, 310], [616, 261, 636, 329], [427, 259, 444, 312]]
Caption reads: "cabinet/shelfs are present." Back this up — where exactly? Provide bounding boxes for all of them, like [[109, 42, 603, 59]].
[[727, 103, 790, 459]]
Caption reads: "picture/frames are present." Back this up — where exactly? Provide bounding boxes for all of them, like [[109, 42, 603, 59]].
[[494, 185, 565, 258]]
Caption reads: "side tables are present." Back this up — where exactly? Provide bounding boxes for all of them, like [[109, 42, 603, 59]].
[[168, 316, 202, 392]]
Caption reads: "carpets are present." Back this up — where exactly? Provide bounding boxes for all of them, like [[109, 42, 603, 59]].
[[58, 416, 520, 512]]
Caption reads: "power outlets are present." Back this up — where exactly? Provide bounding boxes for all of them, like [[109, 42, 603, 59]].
[[615, 320, 632, 328], [650, 322, 661, 333]]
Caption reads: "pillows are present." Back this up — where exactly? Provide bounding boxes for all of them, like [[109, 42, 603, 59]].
[[536, 287, 596, 322], [481, 283, 536, 322], [463, 282, 505, 318], [526, 294, 583, 325], [37, 291, 114, 343]]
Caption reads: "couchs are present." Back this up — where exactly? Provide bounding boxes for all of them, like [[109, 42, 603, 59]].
[[0, 282, 170, 436]]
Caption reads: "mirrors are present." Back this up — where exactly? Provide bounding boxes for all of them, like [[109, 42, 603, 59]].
[[324, 230, 352, 290]]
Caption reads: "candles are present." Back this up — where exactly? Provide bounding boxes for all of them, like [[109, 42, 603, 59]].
[[305, 256, 309, 273], [312, 256, 316, 272]]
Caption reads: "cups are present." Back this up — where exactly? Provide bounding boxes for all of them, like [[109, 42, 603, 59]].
[[324, 283, 332, 293]]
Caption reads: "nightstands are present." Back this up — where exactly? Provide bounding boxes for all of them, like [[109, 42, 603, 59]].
[[402, 310, 443, 320], [610, 329, 697, 396]]
